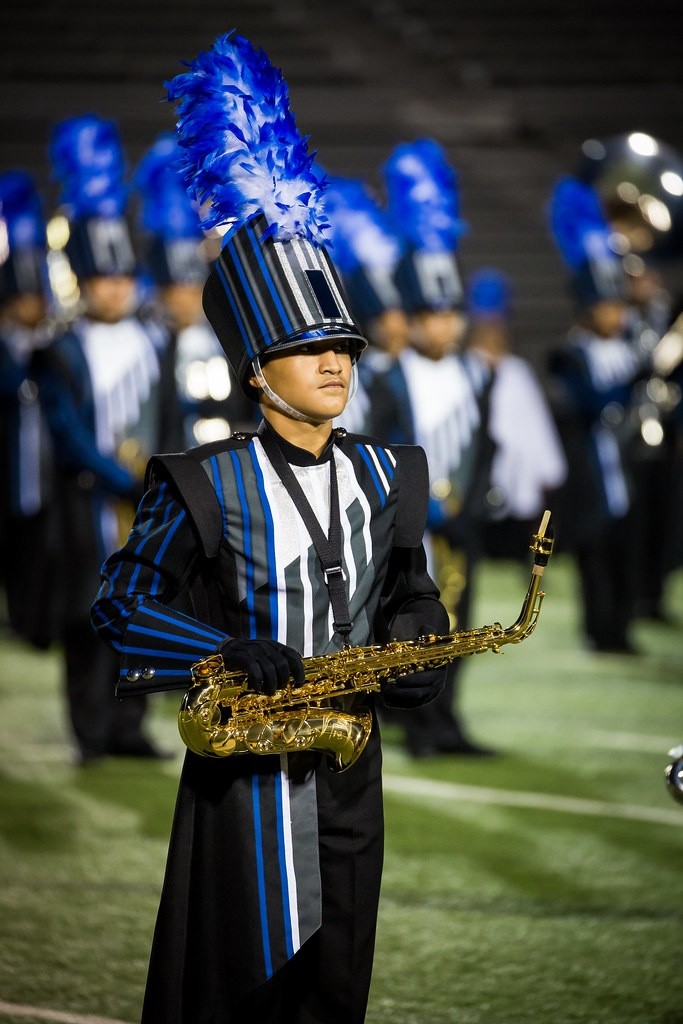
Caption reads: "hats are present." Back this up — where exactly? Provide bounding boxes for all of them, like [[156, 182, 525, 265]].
[[49, 114, 135, 280], [468, 264, 505, 327], [0, 171, 52, 306], [320, 178, 402, 323], [161, 28, 367, 392], [549, 178, 622, 302], [126, 132, 210, 284], [382, 135, 463, 310]]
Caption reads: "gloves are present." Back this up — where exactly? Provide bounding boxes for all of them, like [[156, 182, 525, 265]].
[[385, 625, 447, 708], [219, 638, 305, 696]]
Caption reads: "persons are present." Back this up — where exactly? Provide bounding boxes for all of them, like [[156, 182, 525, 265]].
[[91, 36, 448, 1024], [0, 113, 683, 768]]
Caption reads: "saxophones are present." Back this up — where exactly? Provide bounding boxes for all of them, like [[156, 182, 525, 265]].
[[180, 503, 556, 775]]
